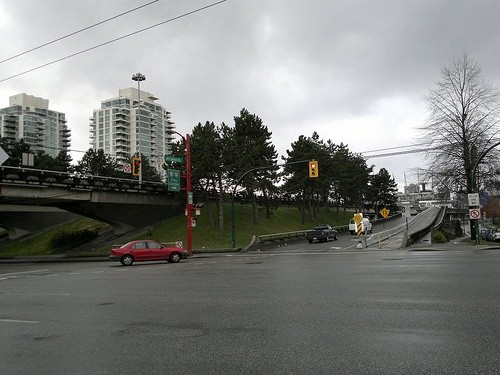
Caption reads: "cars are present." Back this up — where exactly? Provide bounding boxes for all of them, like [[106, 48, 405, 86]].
[[479, 227, 500, 243], [409, 204, 427, 216], [111, 239, 189, 266]]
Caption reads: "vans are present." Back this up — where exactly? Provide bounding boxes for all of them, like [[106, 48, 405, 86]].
[[349, 217, 373, 235]]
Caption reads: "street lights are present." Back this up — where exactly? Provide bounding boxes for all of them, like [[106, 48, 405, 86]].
[[164, 129, 192, 256], [132, 72, 146, 187]]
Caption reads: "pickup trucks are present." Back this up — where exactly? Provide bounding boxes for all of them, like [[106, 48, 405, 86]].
[[305, 224, 338, 244]]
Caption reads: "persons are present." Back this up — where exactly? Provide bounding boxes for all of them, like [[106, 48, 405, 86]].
[[144, 229, 152, 239]]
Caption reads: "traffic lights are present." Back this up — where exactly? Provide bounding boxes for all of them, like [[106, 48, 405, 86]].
[[309, 161, 318, 178], [132, 157, 141, 177]]
